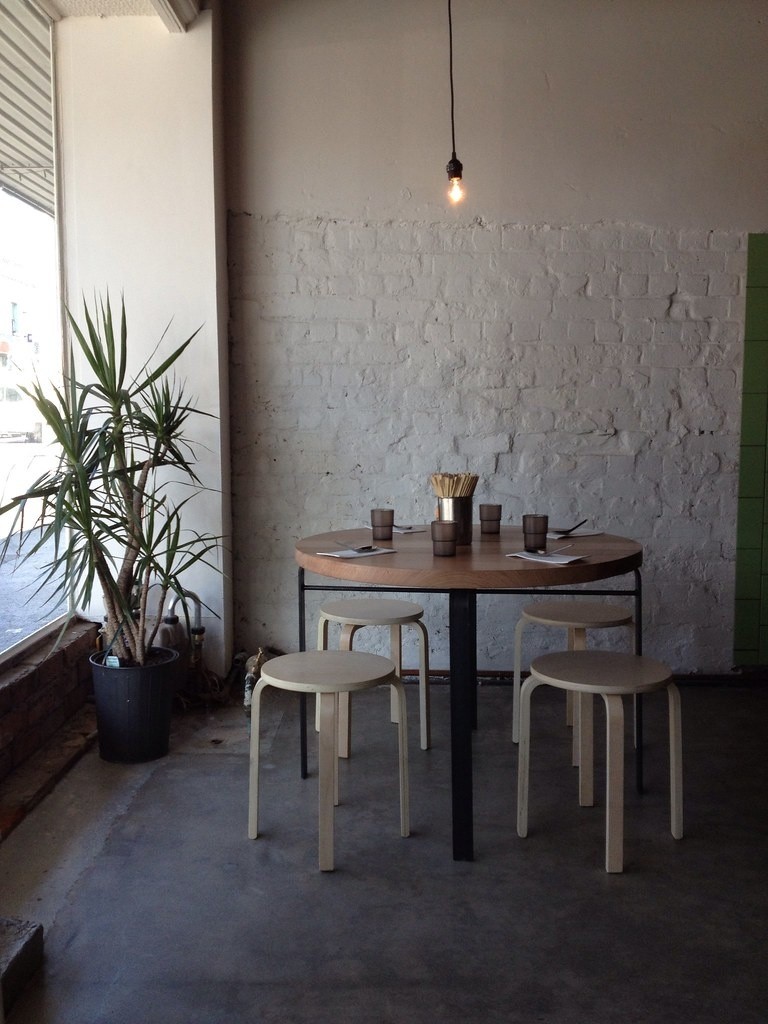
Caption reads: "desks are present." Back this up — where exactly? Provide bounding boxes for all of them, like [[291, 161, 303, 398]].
[[296, 523, 645, 861]]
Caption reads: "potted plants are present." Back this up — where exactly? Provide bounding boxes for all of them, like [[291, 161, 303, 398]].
[[0, 281, 241, 764]]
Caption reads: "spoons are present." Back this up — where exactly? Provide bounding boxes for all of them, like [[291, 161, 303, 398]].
[[538, 544, 574, 556], [393, 525, 412, 530], [335, 541, 378, 553], [552, 519, 588, 535]]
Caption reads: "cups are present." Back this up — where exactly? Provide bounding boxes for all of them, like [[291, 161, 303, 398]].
[[523, 515, 548, 551], [371, 509, 394, 540], [431, 521, 458, 556], [438, 496, 473, 545], [480, 504, 502, 534]]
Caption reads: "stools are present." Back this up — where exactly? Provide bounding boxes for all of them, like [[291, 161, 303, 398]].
[[315, 598, 429, 759], [518, 651, 683, 873], [248, 650, 411, 872], [513, 601, 638, 766]]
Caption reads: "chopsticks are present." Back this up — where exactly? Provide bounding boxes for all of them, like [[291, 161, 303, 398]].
[[430, 472, 480, 497]]
